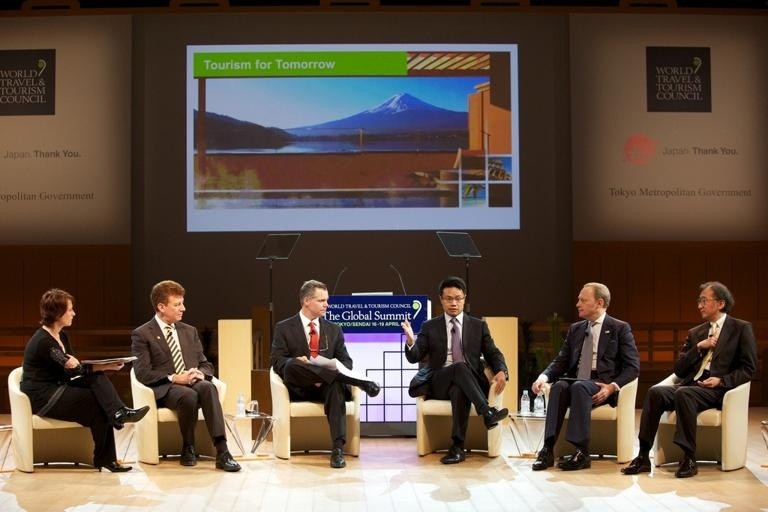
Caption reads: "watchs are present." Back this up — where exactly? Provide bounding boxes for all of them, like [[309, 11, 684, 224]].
[[721, 378, 723, 386], [613, 384, 618, 394]]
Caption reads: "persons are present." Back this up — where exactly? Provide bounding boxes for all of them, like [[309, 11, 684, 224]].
[[131, 280, 241, 472], [620, 281, 758, 478], [268, 279, 380, 468], [532, 282, 641, 471], [400, 276, 509, 464], [20, 288, 150, 472]]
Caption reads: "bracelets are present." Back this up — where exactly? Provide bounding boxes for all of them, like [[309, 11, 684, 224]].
[[172, 376, 175, 385]]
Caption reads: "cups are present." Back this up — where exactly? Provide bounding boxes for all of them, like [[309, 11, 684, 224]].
[[247, 399, 258, 414]]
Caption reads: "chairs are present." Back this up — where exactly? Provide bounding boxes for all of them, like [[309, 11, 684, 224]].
[[7, 365, 101, 473], [222, 411, 280, 459], [415, 357, 502, 459], [539, 375, 640, 465], [130, 362, 229, 465], [648, 370, 752, 473], [268, 363, 364, 461]]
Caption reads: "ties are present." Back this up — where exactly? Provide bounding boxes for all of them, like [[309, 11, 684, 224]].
[[694, 323, 717, 380], [308, 322, 319, 357], [577, 321, 597, 379], [167, 326, 184, 374], [450, 319, 463, 362]]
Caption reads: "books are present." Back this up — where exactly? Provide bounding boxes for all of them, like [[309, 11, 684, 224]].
[[80, 356, 138, 365]]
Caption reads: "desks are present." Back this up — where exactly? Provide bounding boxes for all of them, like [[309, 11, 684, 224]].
[[505, 411, 551, 460], [0, 423, 15, 473], [759, 418, 768, 468]]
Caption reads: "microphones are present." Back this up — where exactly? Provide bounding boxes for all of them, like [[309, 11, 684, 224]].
[[332, 266, 349, 296], [388, 262, 407, 294]]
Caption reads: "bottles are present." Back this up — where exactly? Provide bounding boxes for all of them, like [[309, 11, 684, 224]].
[[520, 390, 530, 414], [237, 392, 245, 417], [534, 389, 545, 413]]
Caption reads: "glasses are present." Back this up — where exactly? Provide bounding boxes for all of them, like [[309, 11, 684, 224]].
[[308, 336, 329, 352], [697, 297, 716, 305], [442, 297, 465, 302]]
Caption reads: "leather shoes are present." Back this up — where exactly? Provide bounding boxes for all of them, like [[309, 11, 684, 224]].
[[532, 448, 554, 469], [360, 381, 379, 397], [621, 456, 651, 475], [559, 451, 591, 470], [330, 449, 345, 468], [484, 407, 508, 429], [113, 406, 150, 430], [440, 447, 465, 463], [216, 451, 241, 471], [98, 461, 132, 473], [181, 446, 197, 466], [676, 458, 698, 477]]
